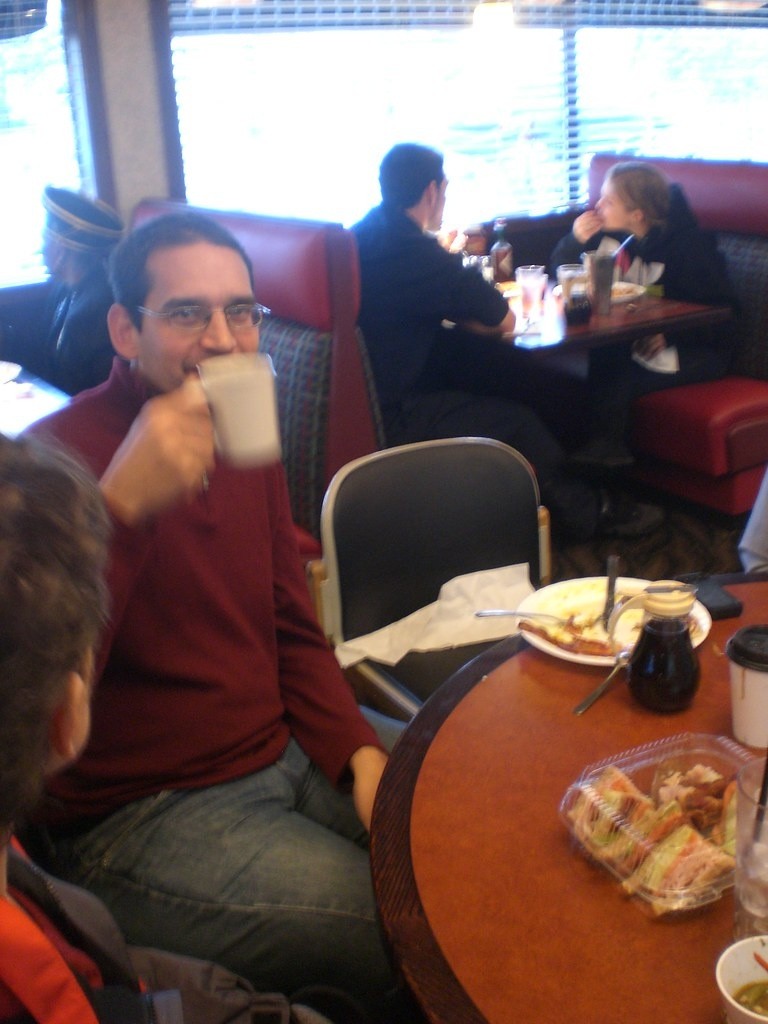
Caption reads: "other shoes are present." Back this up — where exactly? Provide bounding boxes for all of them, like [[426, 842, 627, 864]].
[[596, 493, 666, 538], [570, 442, 634, 468]]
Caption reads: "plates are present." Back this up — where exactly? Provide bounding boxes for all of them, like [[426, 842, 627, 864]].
[[607, 594, 712, 660], [553, 281, 647, 303], [514, 576, 714, 665]]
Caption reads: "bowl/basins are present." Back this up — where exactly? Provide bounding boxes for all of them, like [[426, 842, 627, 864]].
[[716, 935, 768, 1024]]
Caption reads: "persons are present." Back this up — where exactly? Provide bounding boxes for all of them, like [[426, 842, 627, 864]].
[[19, 215, 431, 1024], [546, 164, 743, 476], [349, 146, 664, 539], [39, 189, 118, 398], [738, 465, 768, 575], [0, 434, 328, 1024]]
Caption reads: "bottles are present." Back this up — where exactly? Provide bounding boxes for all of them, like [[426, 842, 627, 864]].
[[491, 218, 513, 282], [608, 577, 699, 713]]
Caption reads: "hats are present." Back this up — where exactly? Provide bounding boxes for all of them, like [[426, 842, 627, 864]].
[[41, 185, 127, 257]]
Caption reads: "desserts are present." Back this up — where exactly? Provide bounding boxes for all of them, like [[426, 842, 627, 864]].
[[571, 764, 739, 915]]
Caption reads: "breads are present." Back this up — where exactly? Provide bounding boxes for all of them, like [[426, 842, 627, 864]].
[[518, 614, 624, 657]]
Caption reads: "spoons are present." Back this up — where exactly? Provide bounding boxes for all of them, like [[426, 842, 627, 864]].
[[571, 641, 636, 714]]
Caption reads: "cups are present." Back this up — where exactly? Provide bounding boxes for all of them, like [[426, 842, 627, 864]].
[[192, 352, 282, 467], [725, 624, 768, 749], [580, 249, 614, 314], [515, 265, 545, 312], [731, 756, 768, 943], [557, 264, 587, 300], [522, 274, 549, 319], [482, 267, 494, 285]]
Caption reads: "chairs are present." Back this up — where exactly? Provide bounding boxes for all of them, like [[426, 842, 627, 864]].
[[310, 437, 554, 713]]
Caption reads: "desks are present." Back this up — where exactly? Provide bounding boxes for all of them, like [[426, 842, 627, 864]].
[[448, 281, 734, 360], [369, 561, 768, 1024]]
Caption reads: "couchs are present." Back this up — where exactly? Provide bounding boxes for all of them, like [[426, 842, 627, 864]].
[[127, 198, 389, 556], [485, 154, 768, 516]]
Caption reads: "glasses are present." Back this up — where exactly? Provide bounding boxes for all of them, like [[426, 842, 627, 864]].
[[128, 303, 270, 336]]
